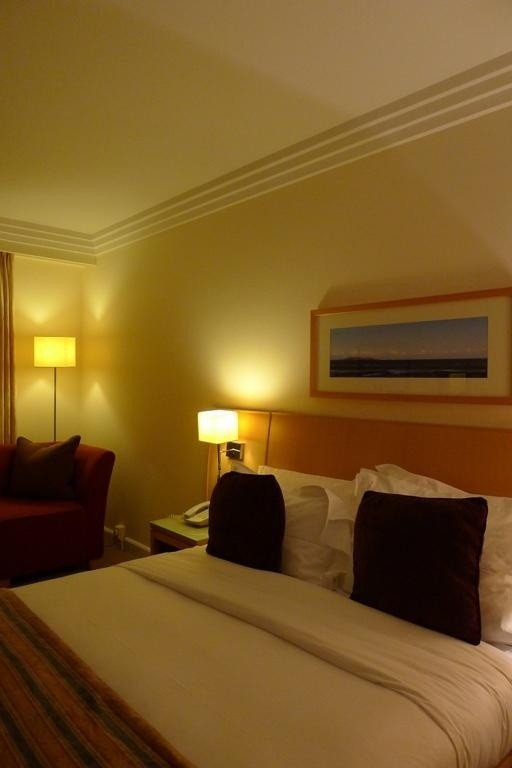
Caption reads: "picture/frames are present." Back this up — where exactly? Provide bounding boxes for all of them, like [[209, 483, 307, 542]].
[[309, 288, 511, 406]]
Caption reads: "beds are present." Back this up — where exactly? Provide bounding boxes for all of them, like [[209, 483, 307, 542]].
[[2, 405, 512, 768]]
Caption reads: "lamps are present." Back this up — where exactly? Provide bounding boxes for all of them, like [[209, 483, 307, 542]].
[[196, 409, 244, 482], [33, 337, 76, 442]]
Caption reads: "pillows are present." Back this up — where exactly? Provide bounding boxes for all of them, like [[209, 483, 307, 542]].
[[207, 471, 286, 574], [231, 463, 352, 601], [355, 469, 512, 651], [373, 463, 456, 492], [12, 436, 85, 499], [349, 490, 489, 646], [255, 464, 355, 504]]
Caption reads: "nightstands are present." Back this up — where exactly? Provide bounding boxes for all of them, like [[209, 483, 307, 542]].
[[149, 514, 209, 557]]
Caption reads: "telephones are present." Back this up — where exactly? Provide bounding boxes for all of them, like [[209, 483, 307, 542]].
[[184, 500, 210, 525]]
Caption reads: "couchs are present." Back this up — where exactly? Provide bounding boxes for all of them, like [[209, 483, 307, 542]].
[[1, 441, 116, 578]]
